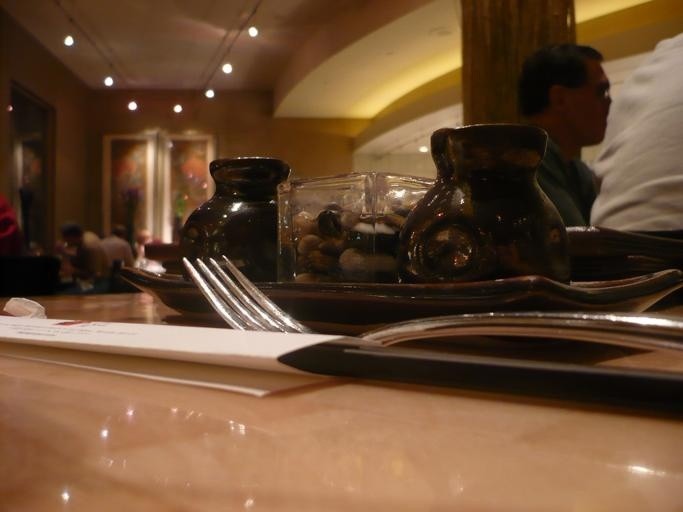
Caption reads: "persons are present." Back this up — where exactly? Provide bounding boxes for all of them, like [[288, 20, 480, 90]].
[[514, 44, 612, 226], [588, 30, 682, 241], [0, 193, 167, 296]]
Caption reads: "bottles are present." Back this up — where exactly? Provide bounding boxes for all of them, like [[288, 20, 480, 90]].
[[190, 155, 289, 277], [396, 126, 568, 283]]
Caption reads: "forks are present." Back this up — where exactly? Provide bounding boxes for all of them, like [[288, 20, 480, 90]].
[[178, 254, 683, 354]]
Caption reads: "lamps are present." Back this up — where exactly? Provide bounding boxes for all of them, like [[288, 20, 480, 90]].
[[54, 0, 144, 113], [199, 0, 263, 99]]
